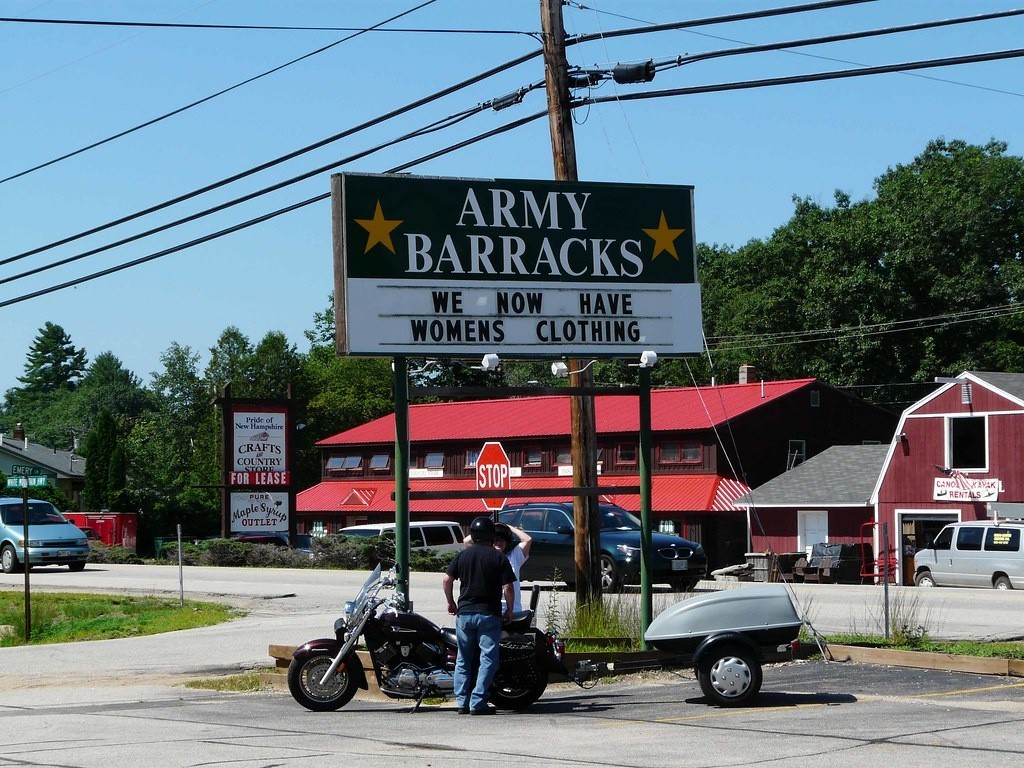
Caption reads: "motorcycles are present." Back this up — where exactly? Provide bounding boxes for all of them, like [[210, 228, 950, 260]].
[[288, 561, 563, 710]]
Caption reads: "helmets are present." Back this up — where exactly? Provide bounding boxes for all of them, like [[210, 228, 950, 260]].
[[470, 516, 495, 542], [494, 521, 512, 542]]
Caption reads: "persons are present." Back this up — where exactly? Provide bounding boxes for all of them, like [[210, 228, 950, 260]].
[[442, 516, 532, 716]]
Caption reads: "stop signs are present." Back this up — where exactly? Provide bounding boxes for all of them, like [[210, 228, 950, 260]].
[[475, 443, 511, 510]]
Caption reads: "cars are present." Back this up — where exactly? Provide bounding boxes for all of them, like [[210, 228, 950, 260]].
[[0, 498, 90, 573]]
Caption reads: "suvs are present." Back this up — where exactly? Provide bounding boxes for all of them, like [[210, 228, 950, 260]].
[[487, 501, 707, 591]]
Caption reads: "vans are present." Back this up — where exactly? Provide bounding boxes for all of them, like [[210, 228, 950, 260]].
[[913, 519, 1024, 590], [338, 521, 466, 560]]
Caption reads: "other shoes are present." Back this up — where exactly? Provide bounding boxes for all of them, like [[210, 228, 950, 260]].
[[469, 706, 495, 715], [458, 707, 469, 714]]
